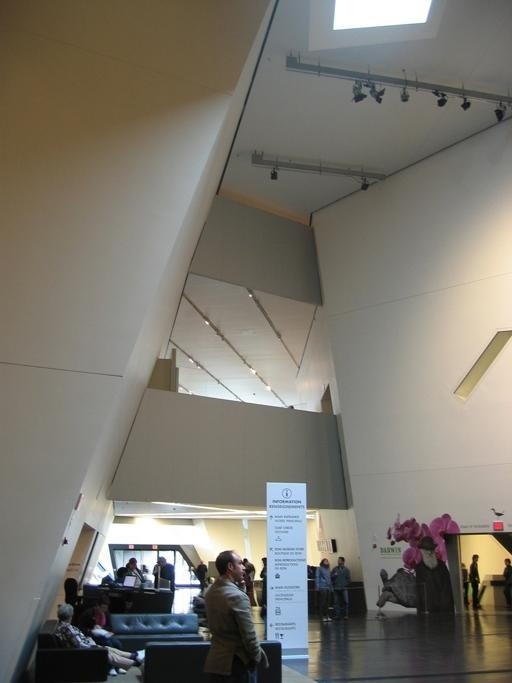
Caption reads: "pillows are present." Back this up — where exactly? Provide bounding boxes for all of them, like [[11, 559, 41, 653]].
[[37, 619, 58, 648]]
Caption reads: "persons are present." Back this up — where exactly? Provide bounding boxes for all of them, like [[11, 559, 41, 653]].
[[79, 595, 127, 676], [242, 558, 255, 579], [469, 553, 481, 608], [201, 550, 266, 683], [55, 603, 145, 669], [315, 557, 333, 622], [501, 558, 512, 607], [115, 567, 128, 583], [330, 555, 352, 620], [196, 559, 208, 592], [126, 557, 145, 583], [154, 555, 175, 615]]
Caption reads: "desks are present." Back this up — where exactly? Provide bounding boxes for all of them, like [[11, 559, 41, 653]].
[[83, 583, 174, 614], [244, 579, 263, 608]]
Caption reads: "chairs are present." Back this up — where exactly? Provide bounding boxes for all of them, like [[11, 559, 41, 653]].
[[35, 648, 108, 683]]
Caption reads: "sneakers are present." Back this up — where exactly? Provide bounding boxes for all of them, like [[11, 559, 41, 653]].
[[108, 646, 146, 676]]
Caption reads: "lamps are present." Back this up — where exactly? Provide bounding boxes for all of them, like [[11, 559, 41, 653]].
[[360, 177, 370, 190], [352, 79, 508, 122], [270, 168, 278, 180]]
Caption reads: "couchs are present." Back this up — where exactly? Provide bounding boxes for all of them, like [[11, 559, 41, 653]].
[[143, 639, 281, 683], [109, 613, 203, 652]]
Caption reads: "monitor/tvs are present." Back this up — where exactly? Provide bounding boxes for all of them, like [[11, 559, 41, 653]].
[[122, 575, 141, 588]]
